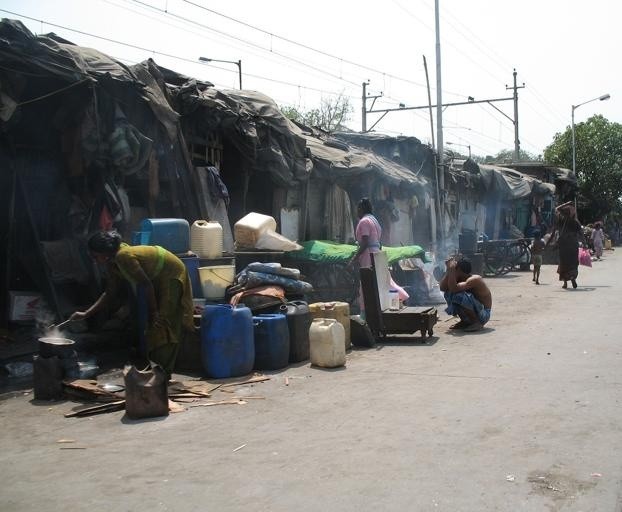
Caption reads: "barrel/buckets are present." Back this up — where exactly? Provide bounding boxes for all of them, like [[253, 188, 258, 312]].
[[275, 301, 311, 362], [196, 266, 236, 300], [201, 303, 255, 379], [178, 255, 199, 298], [249, 312, 291, 371], [308, 318, 347, 369], [131, 217, 191, 255], [189, 220, 224, 259], [309, 300, 352, 350], [233, 212, 277, 250]]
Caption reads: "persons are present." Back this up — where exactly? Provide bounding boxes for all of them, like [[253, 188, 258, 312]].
[[531, 231, 545, 285], [554, 200, 587, 289], [440, 255, 492, 332], [347, 197, 382, 338], [590, 222, 604, 259], [68, 231, 193, 382]]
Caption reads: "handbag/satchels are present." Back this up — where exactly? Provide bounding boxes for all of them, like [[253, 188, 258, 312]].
[[578, 248, 592, 267]]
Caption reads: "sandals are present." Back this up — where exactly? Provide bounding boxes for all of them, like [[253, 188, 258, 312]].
[[449, 321, 482, 331]]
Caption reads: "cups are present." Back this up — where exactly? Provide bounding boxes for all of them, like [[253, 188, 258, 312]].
[[388, 289, 400, 311]]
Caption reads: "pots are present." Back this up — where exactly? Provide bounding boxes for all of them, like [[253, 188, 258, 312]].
[[38, 337, 75, 357]]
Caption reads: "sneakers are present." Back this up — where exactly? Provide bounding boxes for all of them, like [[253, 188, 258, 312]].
[[572, 282, 577, 288], [562, 284, 567, 288]]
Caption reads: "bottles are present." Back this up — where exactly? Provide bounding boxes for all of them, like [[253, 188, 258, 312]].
[[400, 298, 403, 309]]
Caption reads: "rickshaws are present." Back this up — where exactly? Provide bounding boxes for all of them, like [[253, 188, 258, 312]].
[[480, 234, 534, 277]]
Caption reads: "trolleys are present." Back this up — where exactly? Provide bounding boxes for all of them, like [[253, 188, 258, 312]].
[[370, 249, 438, 343]]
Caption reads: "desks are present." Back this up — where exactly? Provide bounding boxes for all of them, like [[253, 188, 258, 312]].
[[381, 305, 437, 343]]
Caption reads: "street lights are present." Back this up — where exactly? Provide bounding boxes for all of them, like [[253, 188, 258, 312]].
[[445, 141, 472, 157], [198, 55, 243, 90], [571, 94, 613, 219]]
